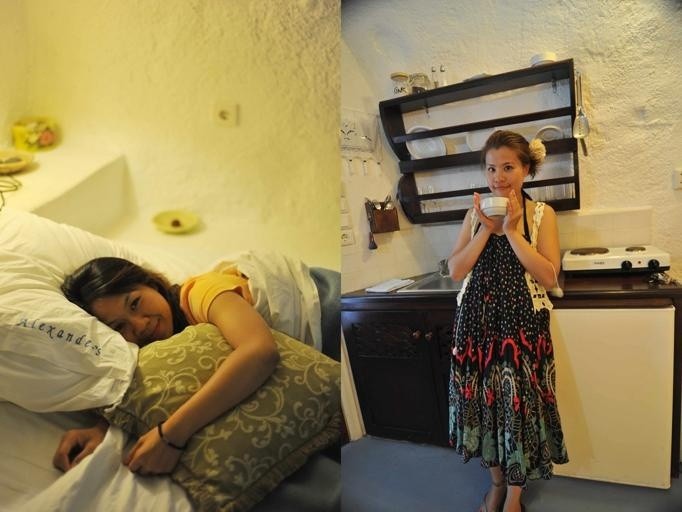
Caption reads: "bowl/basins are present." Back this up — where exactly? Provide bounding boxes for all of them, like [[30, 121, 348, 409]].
[[11, 116, 57, 151]]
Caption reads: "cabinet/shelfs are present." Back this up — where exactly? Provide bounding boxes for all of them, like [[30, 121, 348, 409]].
[[378, 54, 586, 160], [395, 137, 581, 225], [337, 294, 464, 451]]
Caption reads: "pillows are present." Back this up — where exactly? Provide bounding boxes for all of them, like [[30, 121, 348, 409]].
[[1, 200, 147, 416], [96, 320, 342, 511]]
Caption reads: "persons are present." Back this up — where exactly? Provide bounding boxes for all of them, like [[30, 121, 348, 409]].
[[449, 129, 570, 512], [52, 258, 280, 476]]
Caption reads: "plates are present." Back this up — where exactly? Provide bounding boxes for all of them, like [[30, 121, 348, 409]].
[[404, 126, 446, 159], [466, 126, 497, 152], [534, 125, 566, 142], [151, 210, 198, 234], [1, 152, 33, 173]]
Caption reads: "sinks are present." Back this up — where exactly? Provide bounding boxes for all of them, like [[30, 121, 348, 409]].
[[396, 272, 465, 292]]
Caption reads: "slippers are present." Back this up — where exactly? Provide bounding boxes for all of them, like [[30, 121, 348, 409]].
[[479, 491, 527, 512]]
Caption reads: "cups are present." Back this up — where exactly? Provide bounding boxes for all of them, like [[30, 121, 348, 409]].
[[439, 264, 450, 278], [480, 197, 508, 220], [529, 52, 557, 69]]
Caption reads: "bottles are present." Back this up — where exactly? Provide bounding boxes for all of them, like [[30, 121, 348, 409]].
[[391, 64, 447, 96]]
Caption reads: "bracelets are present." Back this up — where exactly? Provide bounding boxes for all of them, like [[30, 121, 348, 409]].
[[158, 421, 188, 450]]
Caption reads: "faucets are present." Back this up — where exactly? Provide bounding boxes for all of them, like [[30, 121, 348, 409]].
[[439, 259, 451, 277]]
